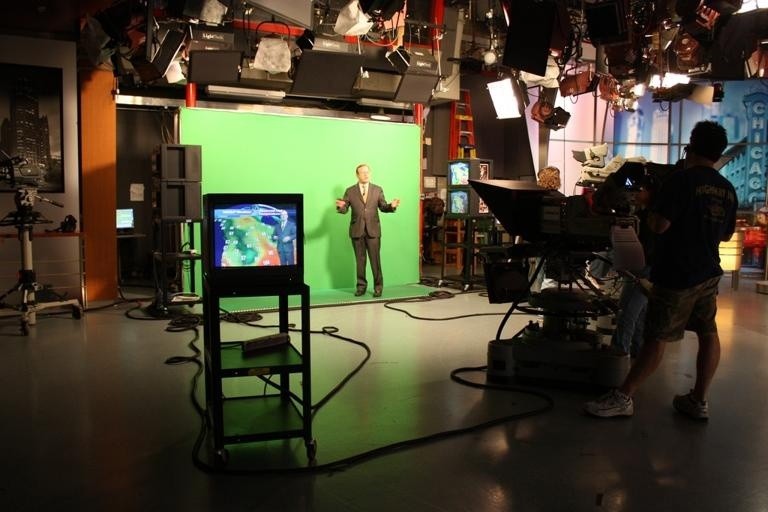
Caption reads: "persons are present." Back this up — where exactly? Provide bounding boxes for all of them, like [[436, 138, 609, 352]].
[[271, 209, 297, 265], [609, 220, 655, 359], [336, 164, 401, 297], [528, 164, 561, 294], [584, 118, 737, 421]]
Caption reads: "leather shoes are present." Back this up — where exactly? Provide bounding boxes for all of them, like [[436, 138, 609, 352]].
[[373, 293, 382, 296], [355, 290, 365, 296]]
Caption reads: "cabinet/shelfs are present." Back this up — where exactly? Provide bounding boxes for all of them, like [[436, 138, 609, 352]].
[[205, 281, 312, 450], [441, 213, 496, 288], [152, 219, 207, 317]]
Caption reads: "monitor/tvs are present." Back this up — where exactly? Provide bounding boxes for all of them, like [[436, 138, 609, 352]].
[[161, 144, 203, 181], [116, 208, 136, 231], [446, 187, 479, 215], [447, 159, 480, 187], [161, 182, 202, 222], [202, 192, 304, 290]]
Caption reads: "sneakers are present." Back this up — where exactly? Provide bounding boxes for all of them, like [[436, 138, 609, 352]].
[[599, 349, 631, 359], [583, 388, 634, 417], [673, 389, 709, 419]]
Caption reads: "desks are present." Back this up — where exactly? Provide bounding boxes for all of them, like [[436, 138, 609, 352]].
[[118, 232, 147, 301]]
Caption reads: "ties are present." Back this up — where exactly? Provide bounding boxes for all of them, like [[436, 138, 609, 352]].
[[362, 185, 367, 203], [282, 221, 284, 231]]
